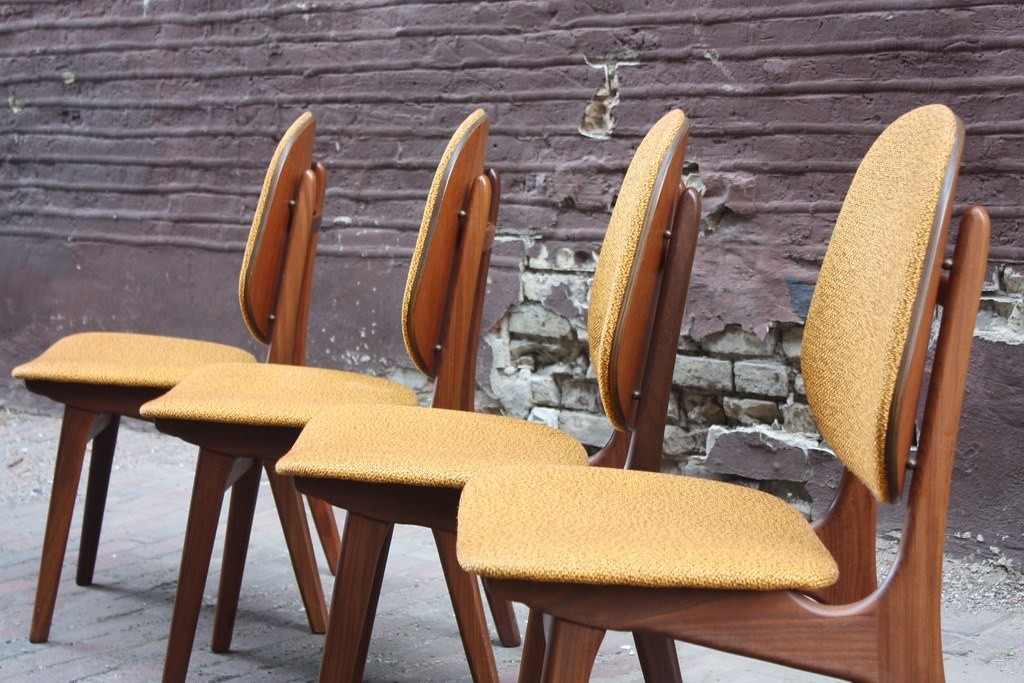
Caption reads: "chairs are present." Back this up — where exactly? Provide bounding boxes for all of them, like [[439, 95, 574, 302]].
[[274, 108, 701, 683], [10, 112, 343, 643], [456, 105, 991, 683], [139, 106, 522, 683]]
